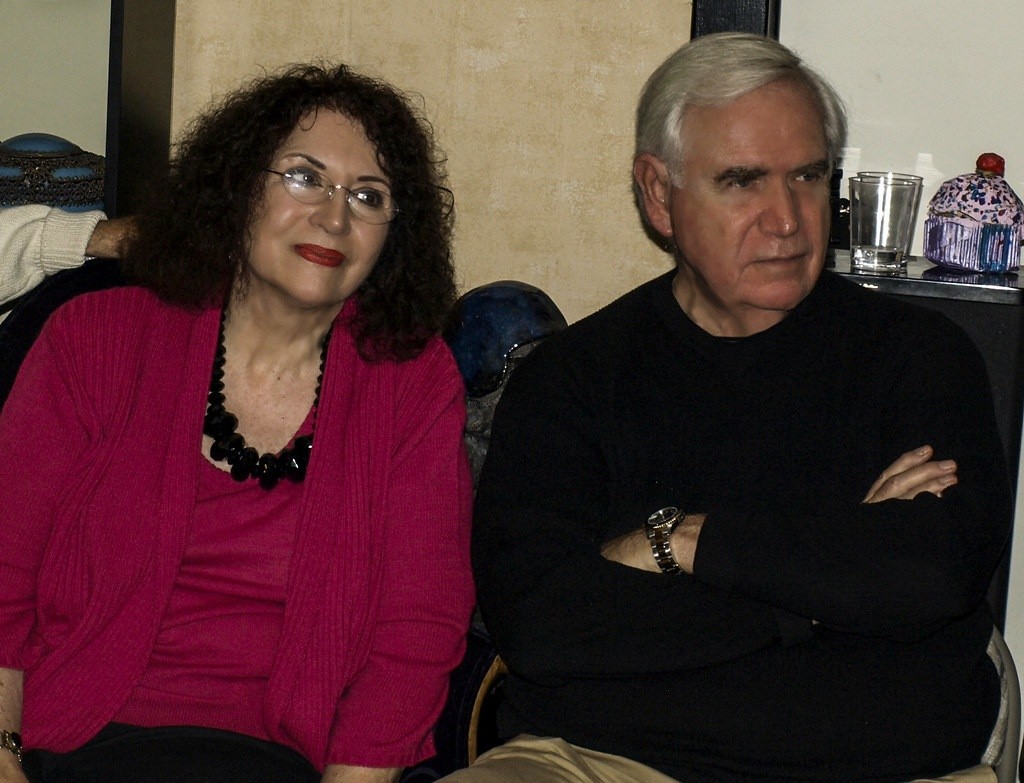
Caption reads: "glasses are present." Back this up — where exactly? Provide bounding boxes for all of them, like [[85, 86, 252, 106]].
[[257, 167, 399, 224]]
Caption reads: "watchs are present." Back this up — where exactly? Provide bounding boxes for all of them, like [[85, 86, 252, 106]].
[[643, 504, 687, 575]]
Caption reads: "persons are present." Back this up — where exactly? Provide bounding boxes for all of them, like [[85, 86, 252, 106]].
[[0, 57, 476, 783], [433, 33, 1011, 783]]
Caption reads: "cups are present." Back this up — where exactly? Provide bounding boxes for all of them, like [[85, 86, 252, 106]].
[[848, 171, 924, 276]]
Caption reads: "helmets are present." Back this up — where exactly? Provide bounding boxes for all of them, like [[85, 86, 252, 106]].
[[442, 279, 569, 400]]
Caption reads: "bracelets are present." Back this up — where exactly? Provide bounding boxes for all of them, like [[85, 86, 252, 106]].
[[0, 730, 22, 765]]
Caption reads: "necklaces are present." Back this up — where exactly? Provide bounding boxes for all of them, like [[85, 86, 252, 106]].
[[203, 304, 334, 489]]
